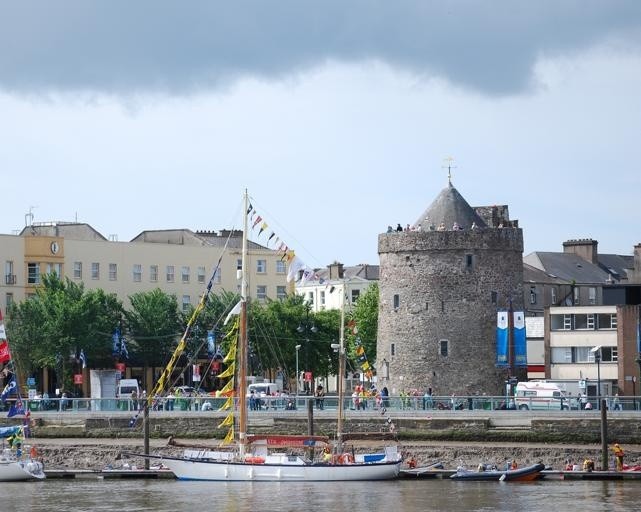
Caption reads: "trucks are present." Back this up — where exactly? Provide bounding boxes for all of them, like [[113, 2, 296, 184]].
[[247, 376, 284, 392], [117, 379, 140, 399], [246, 383, 282, 401]]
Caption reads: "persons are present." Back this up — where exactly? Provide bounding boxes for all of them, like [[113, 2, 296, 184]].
[[352, 385, 389, 409], [615, 444, 624, 466], [512, 460, 518, 470], [407, 457, 416, 469], [42, 392, 50, 409], [562, 392, 621, 411], [249, 390, 295, 411], [315, 384, 325, 410], [566, 459, 573, 470], [130, 390, 213, 411], [378, 204, 523, 234], [399, 388, 473, 410], [58, 392, 68, 411]]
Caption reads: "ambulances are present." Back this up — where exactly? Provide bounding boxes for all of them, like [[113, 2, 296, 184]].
[[515, 380, 572, 410]]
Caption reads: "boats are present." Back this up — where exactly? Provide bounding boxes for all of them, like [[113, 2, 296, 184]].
[[450, 463, 545, 481], [400, 460, 444, 477]]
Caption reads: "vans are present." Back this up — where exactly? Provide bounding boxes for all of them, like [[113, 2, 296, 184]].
[[177, 385, 195, 397]]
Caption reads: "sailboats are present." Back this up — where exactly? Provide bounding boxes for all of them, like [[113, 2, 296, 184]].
[[119, 189, 406, 482], [0, 308, 47, 481]]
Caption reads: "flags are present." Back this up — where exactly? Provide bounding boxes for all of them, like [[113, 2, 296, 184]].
[[0, 310, 26, 418]]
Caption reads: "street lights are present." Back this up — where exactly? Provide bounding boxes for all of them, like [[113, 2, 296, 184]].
[[591, 344, 603, 411], [296, 307, 319, 396]]
